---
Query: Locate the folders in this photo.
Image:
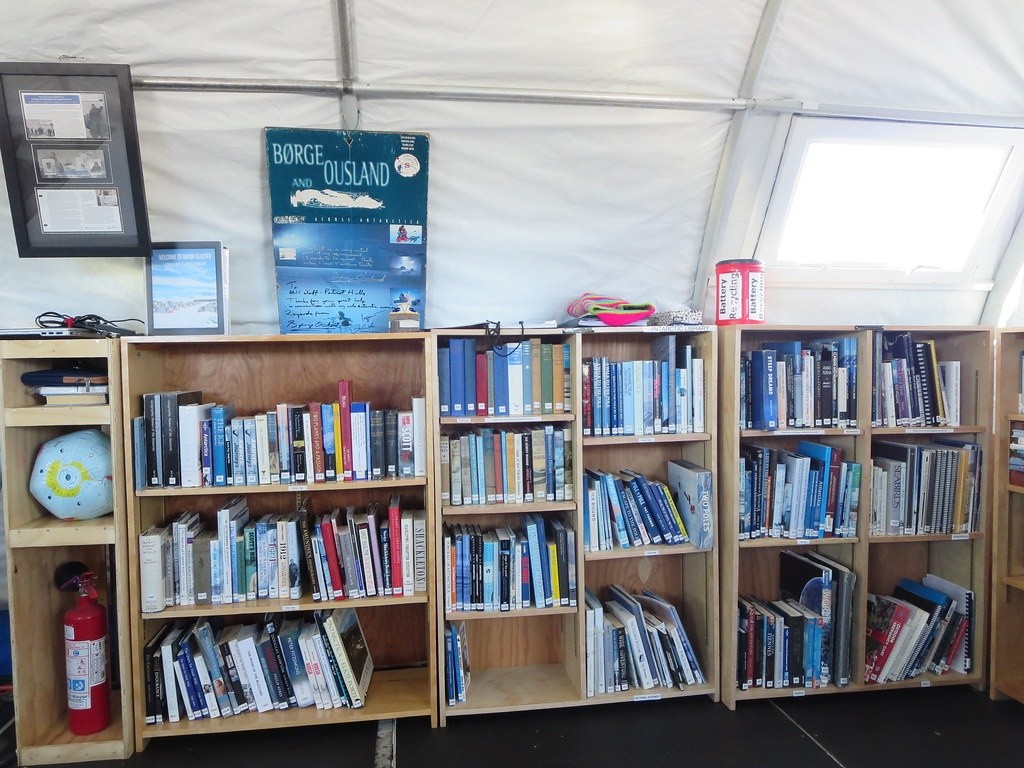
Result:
[438,338,476,416]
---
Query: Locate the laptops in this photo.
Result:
[0,328,136,339]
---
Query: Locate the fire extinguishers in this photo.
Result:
[61,572,112,735]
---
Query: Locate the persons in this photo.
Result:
[27,99,108,178]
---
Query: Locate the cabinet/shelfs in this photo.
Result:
[119,331,438,752]
[0,337,134,767]
[718,324,994,711]
[990,327,1024,704]
[429,324,730,728]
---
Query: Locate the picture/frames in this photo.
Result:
[145,241,224,336]
[0,62,153,258]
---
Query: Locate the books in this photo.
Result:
[868,438,984,538]
[439,334,572,417]
[442,512,578,615]
[865,571,974,685]
[1018,351,1024,413]
[144,607,376,727]
[737,549,854,692]
[583,457,716,553]
[1008,426,1024,488]
[137,491,428,613]
[739,438,862,545]
[439,422,574,504]
[582,334,710,438]
[38,385,109,407]
[133,374,427,488]
[737,333,859,431]
[584,583,705,698]
[420,318,558,331]
[445,620,471,708]
[872,328,964,429]
[556,312,653,329]
